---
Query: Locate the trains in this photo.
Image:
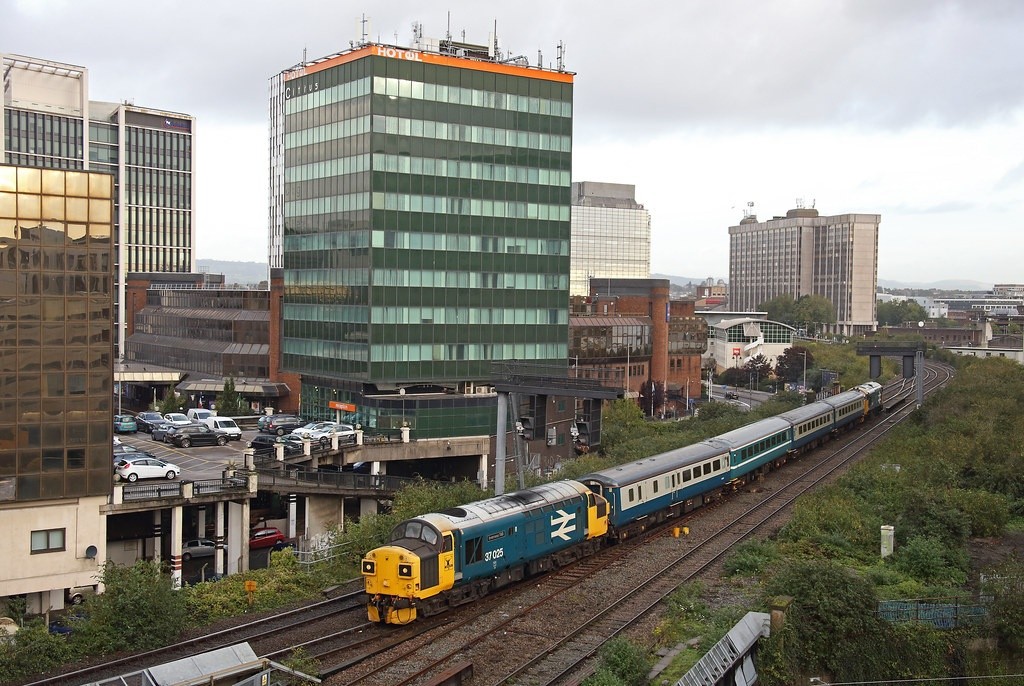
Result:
[359,381,883,626]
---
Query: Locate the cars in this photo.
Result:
[114,410,362,483]
[181,541,228,562]
[249,527,286,550]
